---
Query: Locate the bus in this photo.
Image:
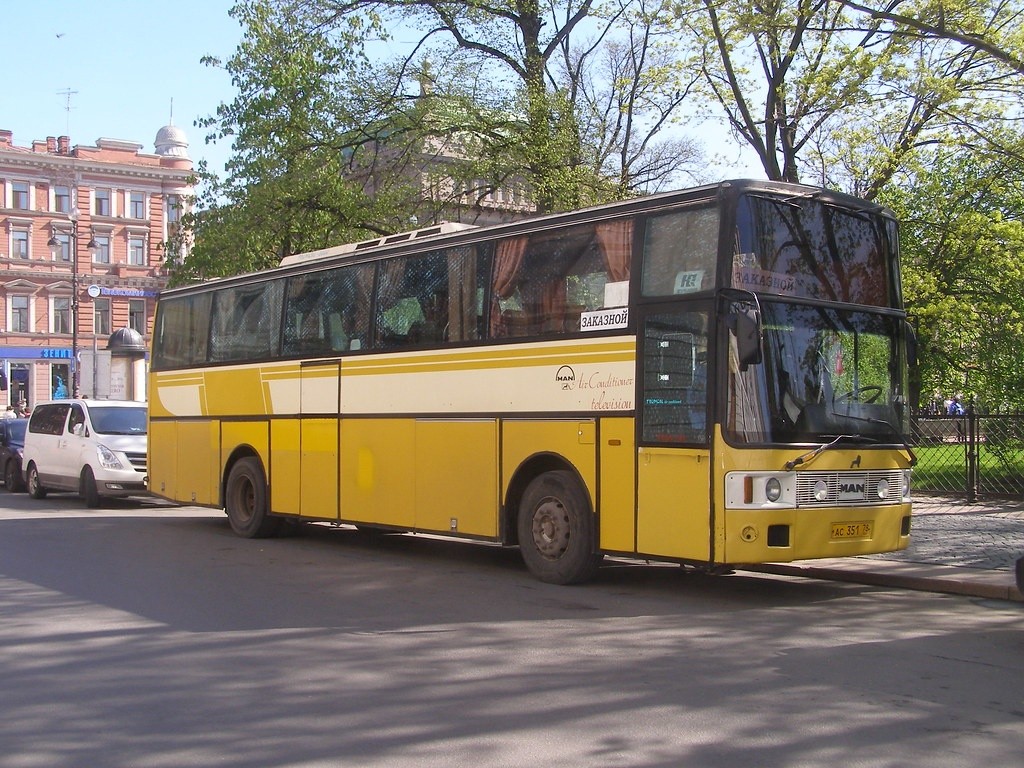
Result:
[144,179,921,586]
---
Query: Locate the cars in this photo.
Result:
[0,419,27,494]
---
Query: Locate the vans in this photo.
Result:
[22,400,148,507]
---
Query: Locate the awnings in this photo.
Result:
[107,327,149,352]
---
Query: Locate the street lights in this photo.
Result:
[46,206,102,400]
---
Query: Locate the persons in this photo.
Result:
[948,397,963,415]
[3,398,28,418]
